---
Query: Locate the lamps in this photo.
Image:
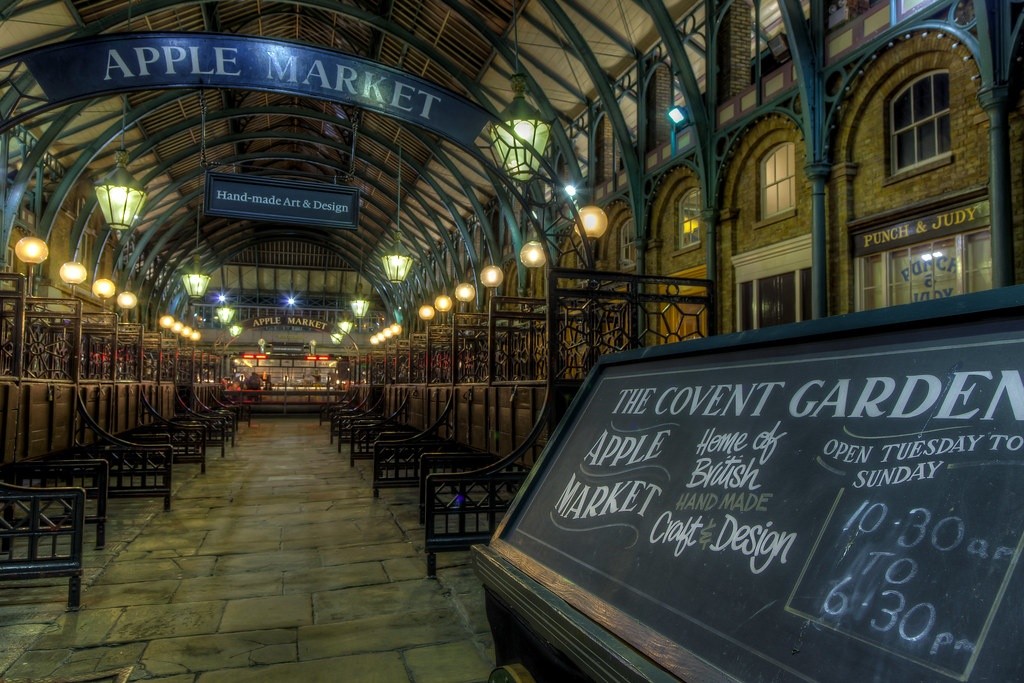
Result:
[419,304,435,332]
[349,229,371,319]
[383,327,394,343]
[337,277,355,335]
[390,323,402,339]
[455,282,476,313]
[189,331,201,347]
[171,321,183,340]
[572,205,607,267]
[480,265,504,297]
[490,1,556,181]
[92,93,150,230]
[668,105,688,126]
[182,126,213,298]
[160,315,174,338]
[228,322,243,337]
[330,333,343,345]
[92,279,116,313]
[521,240,547,297]
[180,325,192,346]
[381,135,414,283]
[60,261,87,299]
[216,261,236,323]
[14,237,49,297]
[434,294,453,322]
[117,290,137,323]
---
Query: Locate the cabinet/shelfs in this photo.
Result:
[322,268,718,581]
[0,272,252,611]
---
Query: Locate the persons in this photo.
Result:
[250,372,272,401]
